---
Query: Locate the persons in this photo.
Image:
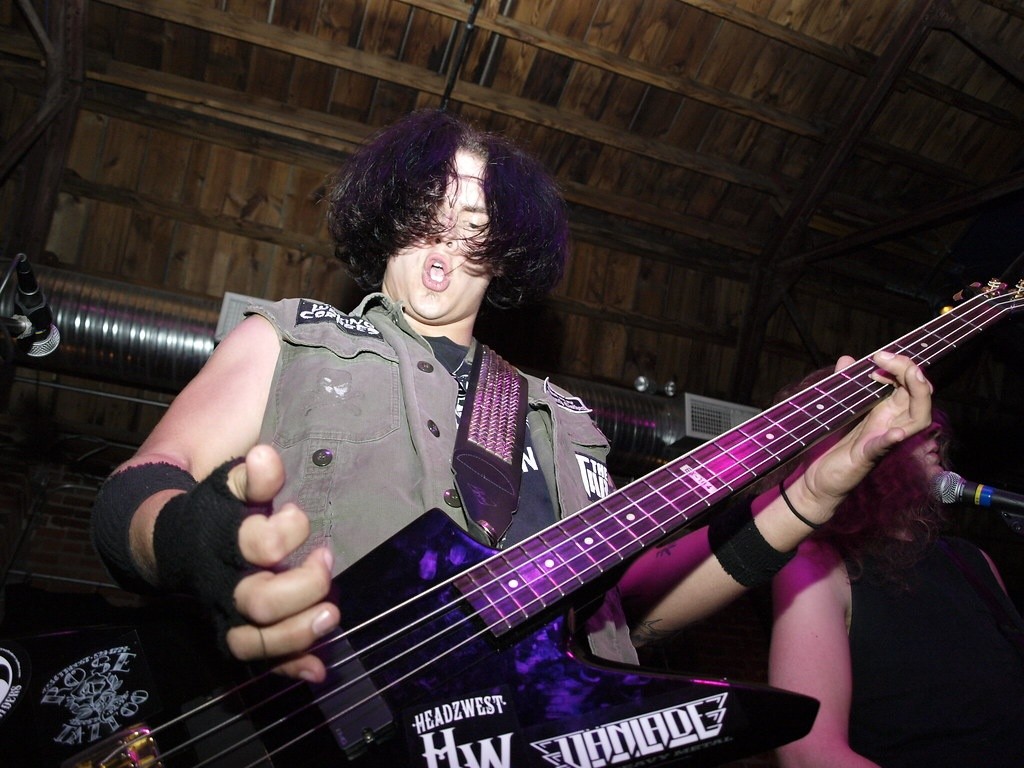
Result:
[769,406,1023,768]
[89,115,1024,768]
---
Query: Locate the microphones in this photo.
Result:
[930,471,1024,518]
[16,252,60,357]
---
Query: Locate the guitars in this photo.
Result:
[0,277,1024,768]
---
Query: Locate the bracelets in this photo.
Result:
[707,499,798,591]
[92,461,198,591]
[779,478,823,530]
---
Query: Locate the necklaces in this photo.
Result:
[451,357,468,392]
